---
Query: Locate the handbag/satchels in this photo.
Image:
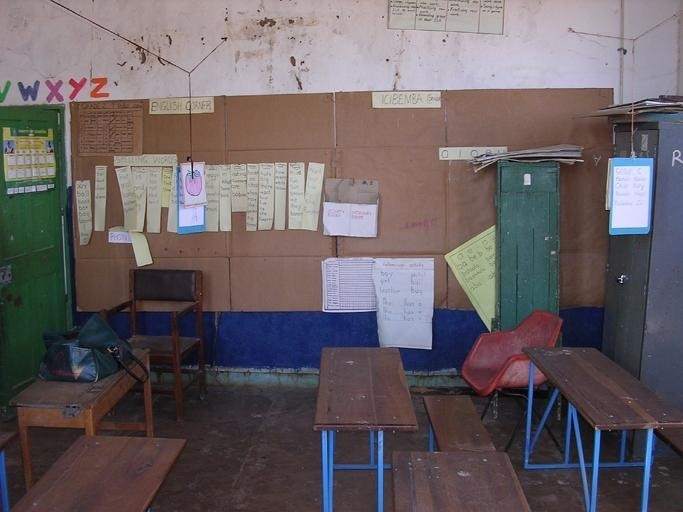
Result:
[35,312,153,385]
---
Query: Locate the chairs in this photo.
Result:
[97,268,210,423]
[459,310,567,456]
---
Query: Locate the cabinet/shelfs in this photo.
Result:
[602,110,681,405]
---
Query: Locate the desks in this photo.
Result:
[309,346,420,512]
[5,347,188,512]
[521,347,682,512]
[393,452,529,510]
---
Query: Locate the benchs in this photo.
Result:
[421,395,496,452]
